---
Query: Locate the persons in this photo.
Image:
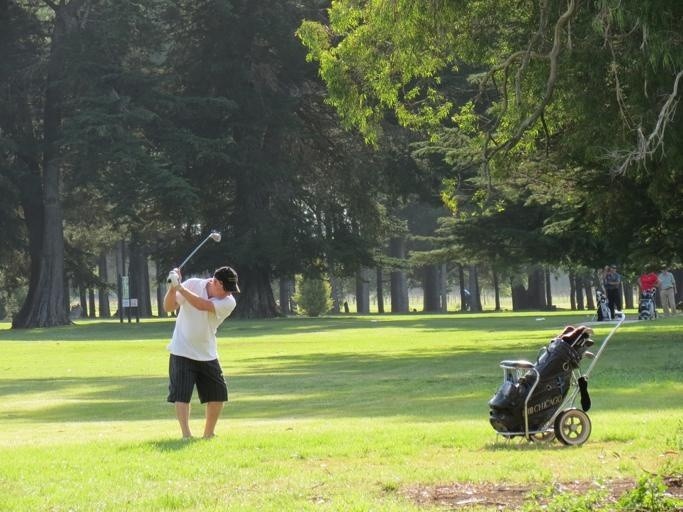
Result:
[637,265,662,292]
[603,264,624,321]
[163,266,240,440]
[658,266,678,318]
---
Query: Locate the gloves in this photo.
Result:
[169,268,182,286]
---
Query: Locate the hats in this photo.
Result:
[214,267,240,294]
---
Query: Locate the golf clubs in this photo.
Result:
[167,232,222,284]
[555,325,596,361]
